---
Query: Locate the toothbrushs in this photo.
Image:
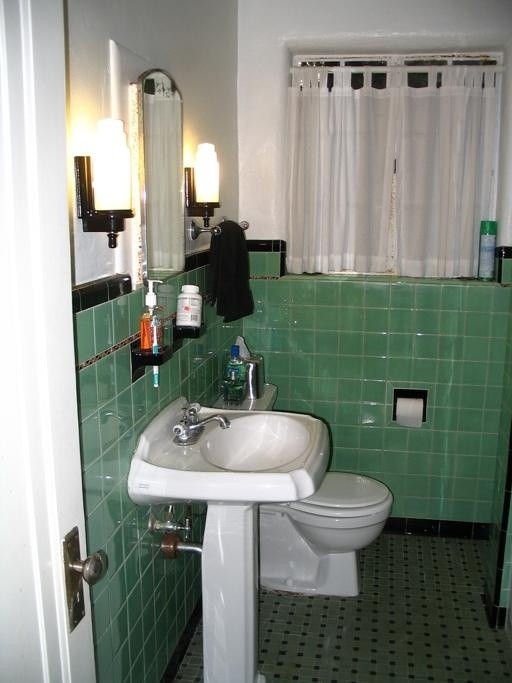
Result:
[151,316,159,387]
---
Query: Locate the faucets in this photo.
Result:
[189,414,230,430]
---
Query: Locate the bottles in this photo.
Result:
[176,284,203,328]
[224,345,247,401]
[157,284,176,327]
[218,368,246,406]
[477,220,497,283]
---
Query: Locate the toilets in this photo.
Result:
[213,382,393,597]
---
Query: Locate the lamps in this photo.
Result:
[182,142,222,229]
[74,119,135,249]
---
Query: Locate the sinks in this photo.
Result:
[127,395,330,504]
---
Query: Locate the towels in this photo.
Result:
[206,220,254,323]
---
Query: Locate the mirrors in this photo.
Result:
[129,68,187,286]
